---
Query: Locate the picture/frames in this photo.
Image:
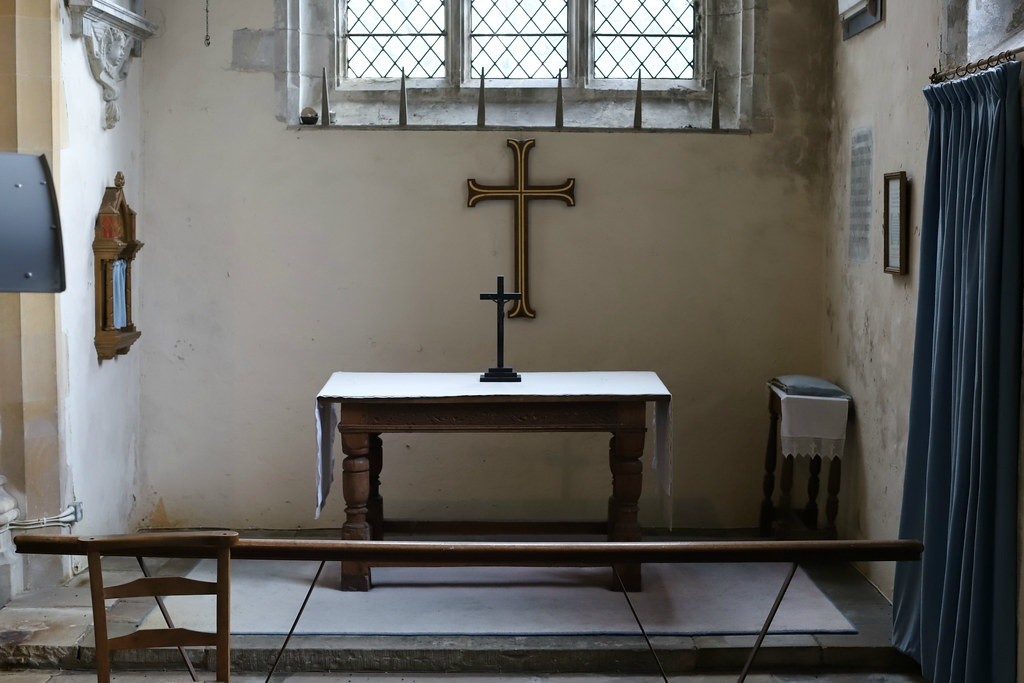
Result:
[883,171,909,276]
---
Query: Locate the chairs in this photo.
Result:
[78,531,241,683]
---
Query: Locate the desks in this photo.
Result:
[761,381,848,564]
[315,371,674,592]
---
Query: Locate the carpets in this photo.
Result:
[141,556,857,635]
